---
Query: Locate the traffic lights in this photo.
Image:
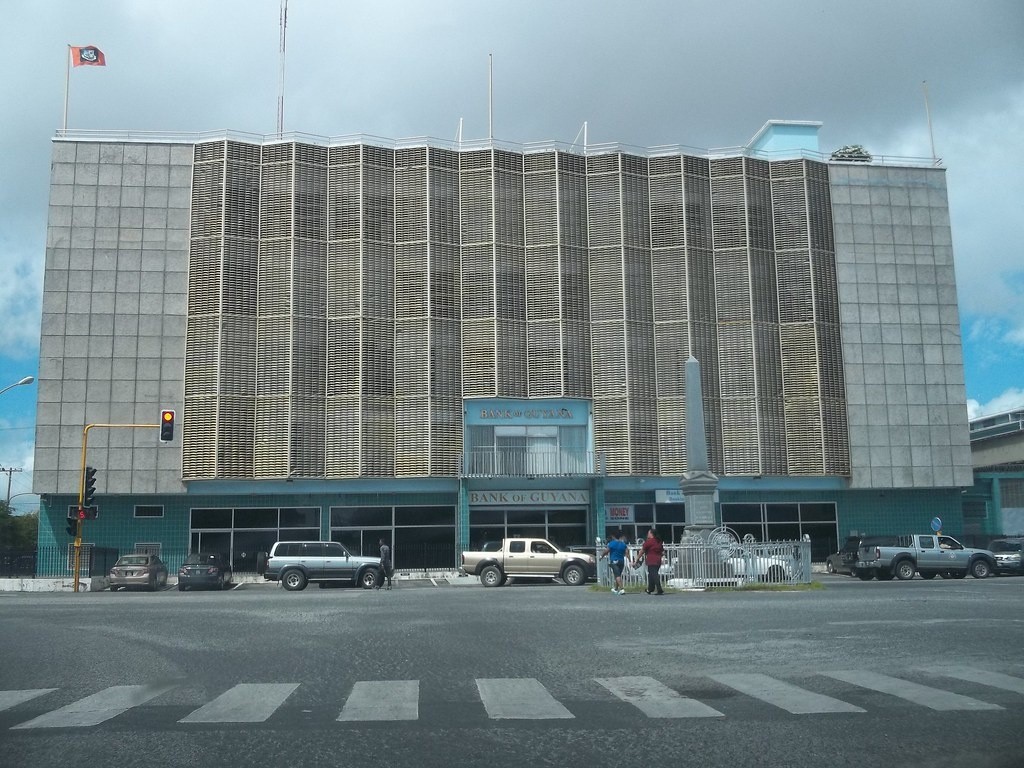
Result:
[83,467,96,508]
[160,410,173,441]
[66,516,78,535]
[80,508,98,519]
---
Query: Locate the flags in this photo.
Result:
[70,45,106,68]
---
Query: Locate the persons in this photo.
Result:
[373,539,392,590]
[633,529,666,595]
[598,530,632,595]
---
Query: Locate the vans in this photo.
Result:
[991,538,1024,575]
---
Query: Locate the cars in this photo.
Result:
[726,547,790,581]
[108,553,168,591]
[178,553,233,591]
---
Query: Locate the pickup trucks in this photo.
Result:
[462,538,597,587]
[874,535,996,580]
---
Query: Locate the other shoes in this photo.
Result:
[645,589,651,594]
[655,591,664,595]
[610,589,618,592]
[385,587,391,590]
[618,589,625,595]
[372,586,379,590]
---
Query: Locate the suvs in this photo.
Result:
[825,536,897,576]
[265,540,393,590]
